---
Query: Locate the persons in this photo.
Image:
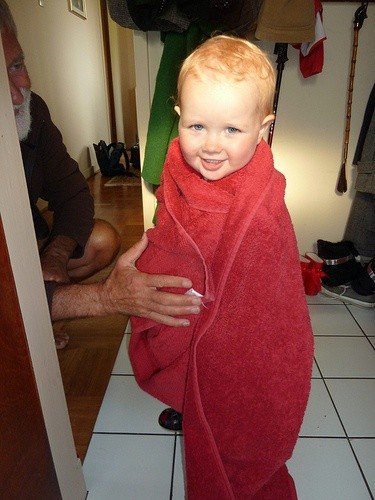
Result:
[136,35,277,427]
[0,0,202,327]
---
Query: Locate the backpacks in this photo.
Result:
[93,140,136,177]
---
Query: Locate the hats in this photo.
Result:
[255,0,316,43]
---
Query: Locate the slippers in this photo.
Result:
[159,408,183,431]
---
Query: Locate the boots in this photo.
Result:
[300,252,327,296]
